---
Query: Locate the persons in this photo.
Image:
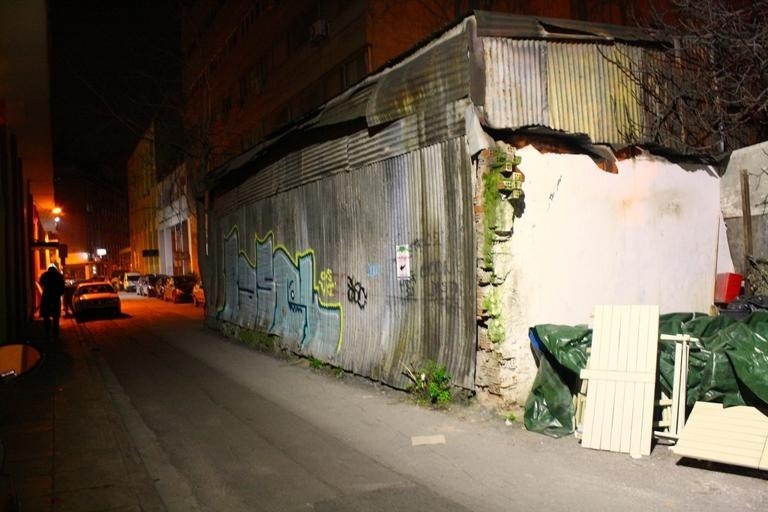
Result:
[39,267,66,338]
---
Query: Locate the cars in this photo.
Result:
[71,281,121,318]
[136,274,205,306]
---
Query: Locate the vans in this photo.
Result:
[123,273,140,292]
